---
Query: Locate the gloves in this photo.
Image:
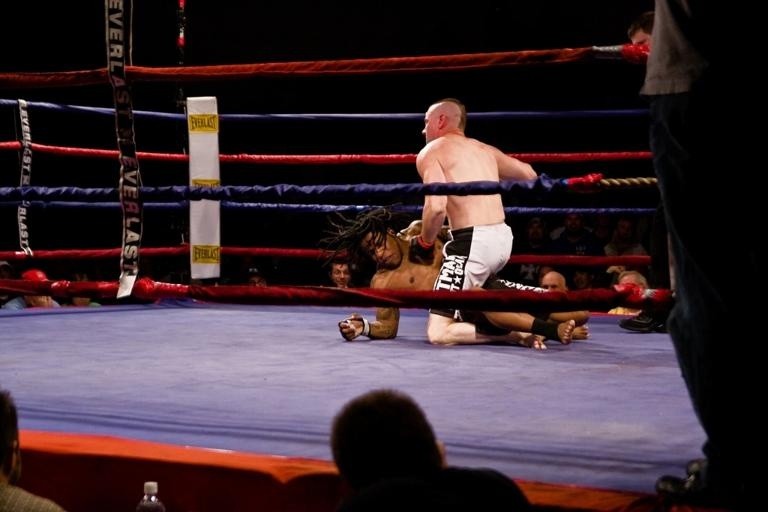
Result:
[409,235,435,266]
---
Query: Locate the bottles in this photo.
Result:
[136,479,165,512]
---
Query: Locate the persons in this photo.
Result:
[324,202,590,346]
[640,0,767,512]
[1,259,101,311]
[330,389,535,512]
[506,202,646,316]
[415,100,547,349]
[249,274,268,289]
[329,262,351,288]
[0,392,65,511]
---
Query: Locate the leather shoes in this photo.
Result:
[619,310,667,335]
[654,458,708,508]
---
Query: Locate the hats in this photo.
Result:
[21,269,49,282]
